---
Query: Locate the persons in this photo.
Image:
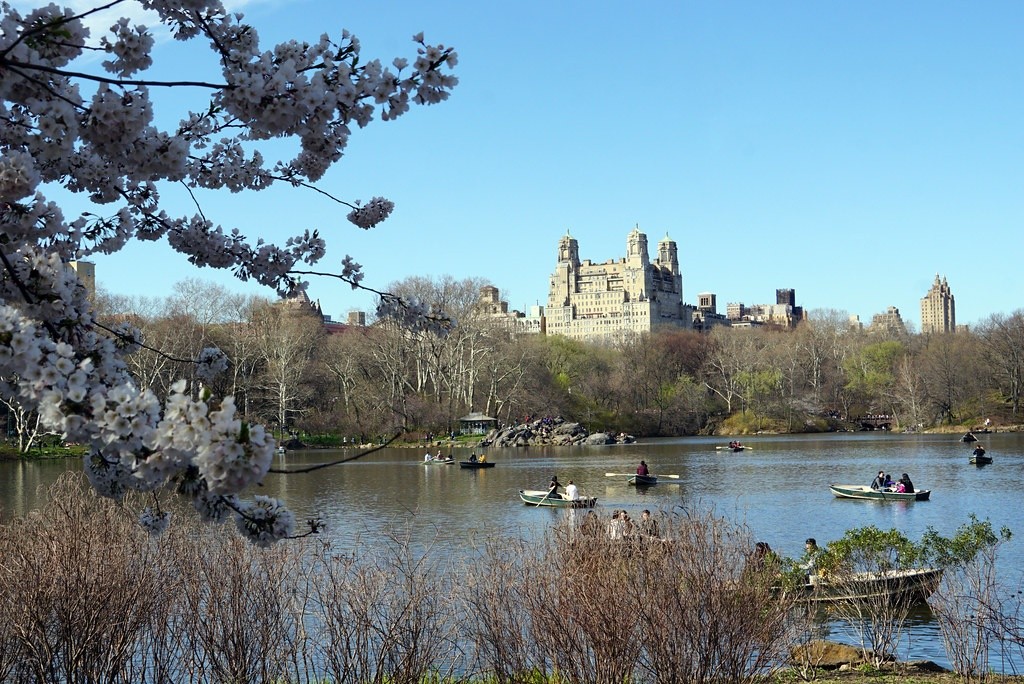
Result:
[289,429,299,439]
[450,430,455,440]
[984,418,990,427]
[359,434,363,445]
[342,436,347,446]
[872,471,915,493]
[582,510,659,540]
[965,432,972,437]
[729,440,741,448]
[435,450,443,459]
[424,452,432,462]
[279,445,284,450]
[515,417,519,424]
[973,445,985,457]
[636,461,649,475]
[564,480,579,500]
[571,426,586,437]
[469,452,477,462]
[542,414,554,435]
[478,454,487,463]
[790,538,824,583]
[751,542,782,586]
[351,437,356,445]
[425,431,434,443]
[970,427,987,432]
[549,476,563,499]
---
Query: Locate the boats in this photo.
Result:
[969,455,993,464]
[828,484,931,501]
[519,489,598,508]
[626,474,657,486]
[459,461,496,468]
[723,446,745,452]
[722,566,946,610]
[419,459,455,465]
[278,448,287,453]
[960,431,979,443]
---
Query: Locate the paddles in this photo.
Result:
[605,472,680,479]
[884,487,898,492]
[959,433,967,441]
[716,446,753,450]
[536,486,555,507]
[969,433,979,441]
[421,455,436,465]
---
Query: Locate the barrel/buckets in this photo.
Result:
[809,575,820,583]
